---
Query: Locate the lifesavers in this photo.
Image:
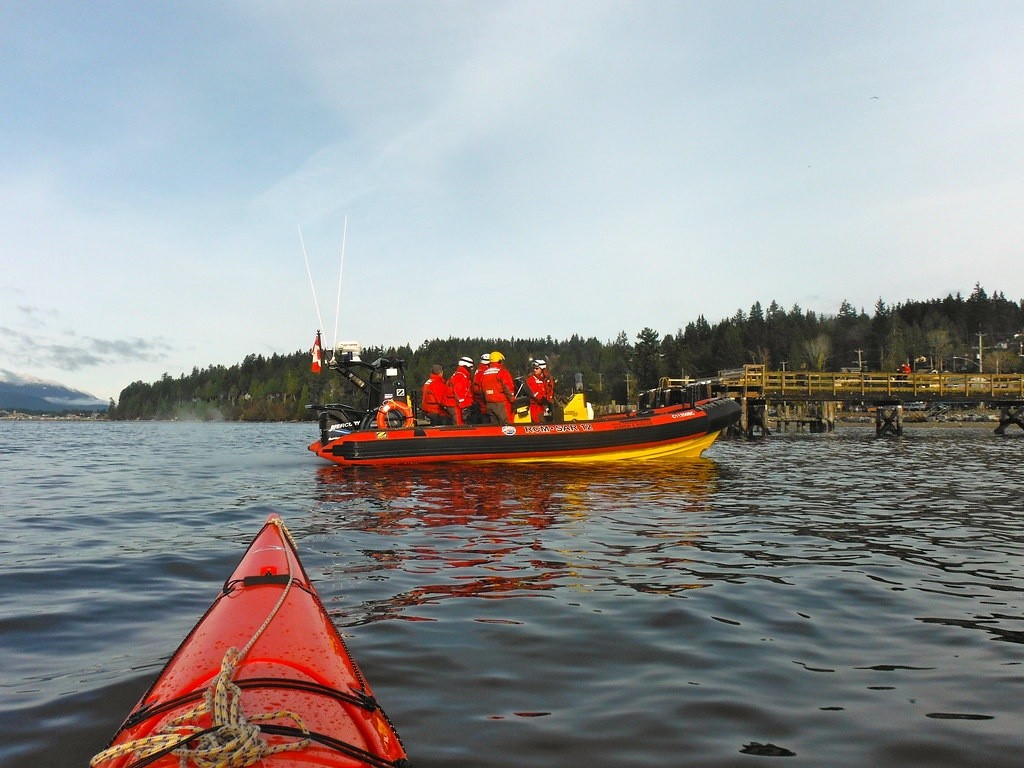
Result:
[376,400,414,429]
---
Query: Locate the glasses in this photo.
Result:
[533,367,540,370]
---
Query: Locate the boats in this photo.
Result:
[296,214,743,471]
[86,511,413,768]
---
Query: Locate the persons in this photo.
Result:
[477,351,515,424]
[524,360,554,424]
[442,357,476,425]
[901,362,911,387]
[473,353,490,424]
[421,365,452,426]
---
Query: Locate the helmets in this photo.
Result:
[479,353,490,364]
[531,359,547,369]
[489,351,505,362]
[458,356,473,367]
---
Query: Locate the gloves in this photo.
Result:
[541,397,548,406]
[547,403,552,414]
[510,402,517,414]
